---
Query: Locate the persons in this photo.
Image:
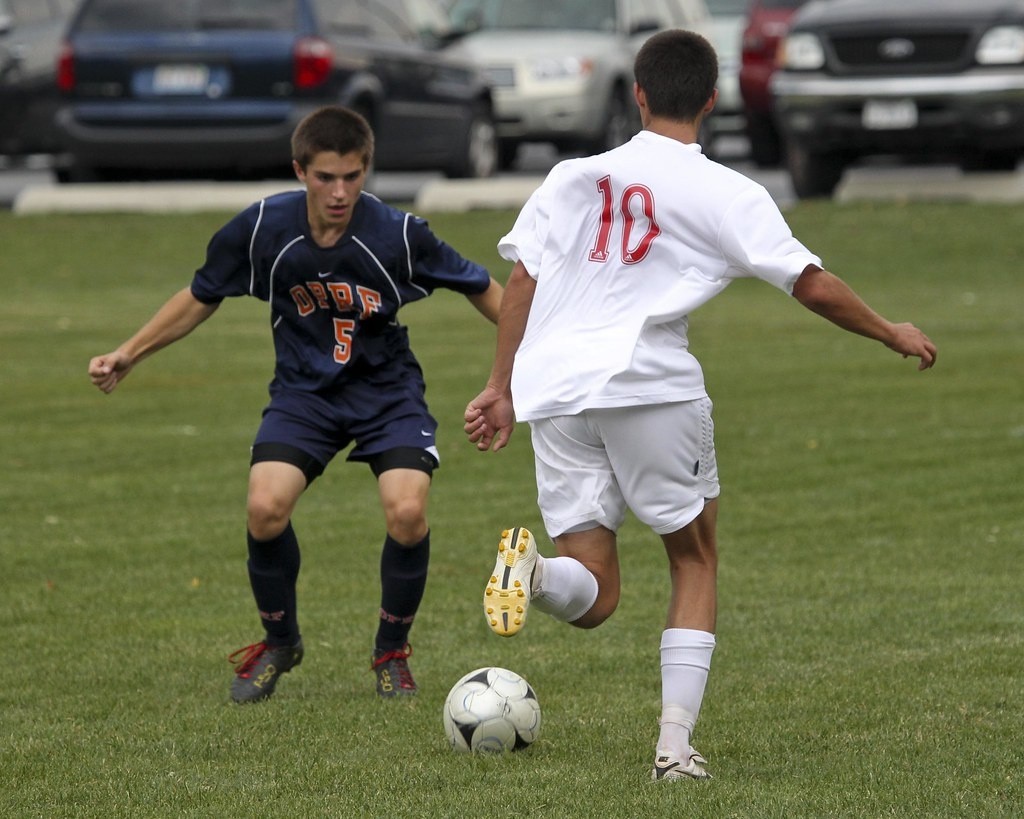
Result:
[462,31,940,781]
[86,105,506,706]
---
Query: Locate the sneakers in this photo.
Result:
[231,637,304,704]
[651,746,714,782]
[371,647,419,697]
[483,527,536,637]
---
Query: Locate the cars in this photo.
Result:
[49,1,500,179]
[742,1,802,158]
[775,0,1024,201]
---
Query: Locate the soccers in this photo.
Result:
[443,667,541,753]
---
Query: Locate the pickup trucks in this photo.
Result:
[450,1,749,172]
